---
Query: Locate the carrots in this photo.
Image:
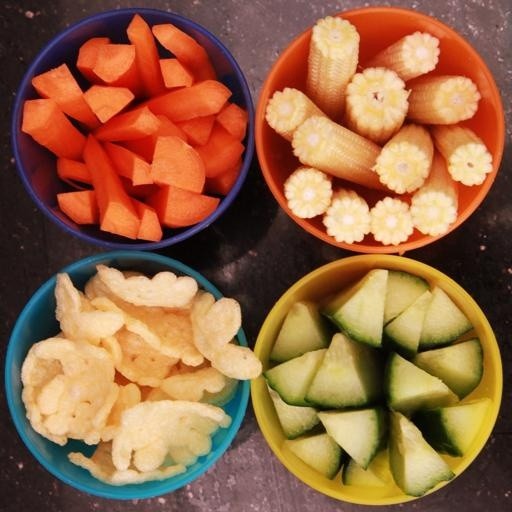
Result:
[21,13,249,244]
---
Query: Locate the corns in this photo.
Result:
[266,15,493,247]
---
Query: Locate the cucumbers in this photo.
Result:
[261,268,491,497]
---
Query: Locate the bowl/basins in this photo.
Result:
[253,5,507,254]
[10,5,256,252]
[4,250,254,504]
[250,254,505,507]
